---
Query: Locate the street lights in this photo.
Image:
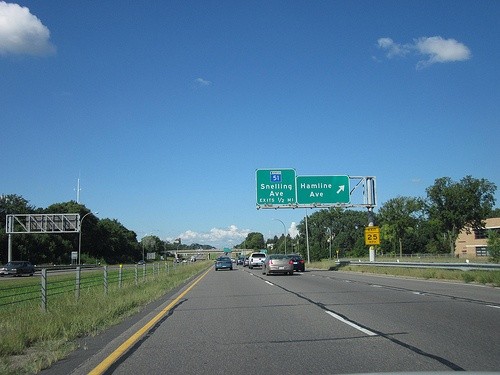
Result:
[272,218,287,255]
[78,211,99,269]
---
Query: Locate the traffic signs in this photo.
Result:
[296,176,350,204]
[255,168,297,206]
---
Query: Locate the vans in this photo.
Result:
[248,252,266,271]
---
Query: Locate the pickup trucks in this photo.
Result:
[214,256,233,271]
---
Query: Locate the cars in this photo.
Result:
[261,255,295,276]
[137,256,202,266]
[286,254,305,272]
[231,256,249,268]
[0,260,35,278]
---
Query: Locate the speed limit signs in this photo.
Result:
[365,226,380,245]
